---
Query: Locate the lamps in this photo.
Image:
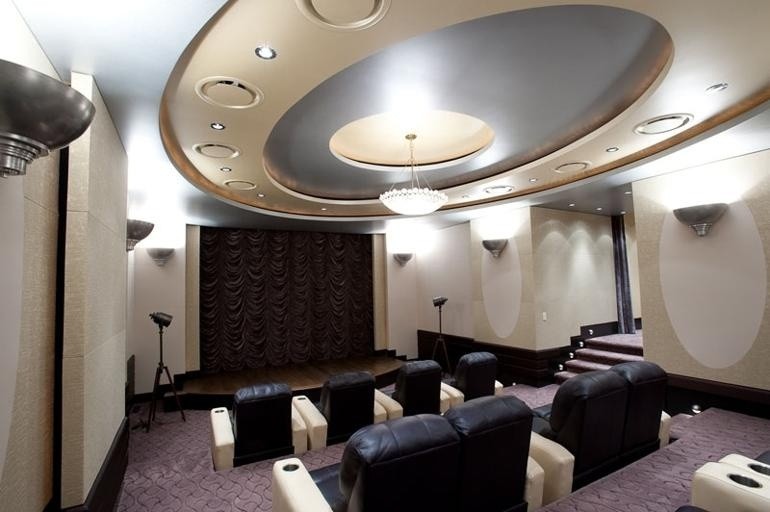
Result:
[431,295,448,308]
[147,311,175,328]
[0,58,97,179]
[394,254,412,267]
[378,132,449,216]
[482,240,509,258]
[127,219,155,250]
[673,203,731,235]
[147,248,174,266]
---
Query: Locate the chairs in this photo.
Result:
[440,352,504,410]
[208,381,308,473]
[292,370,389,450]
[374,359,452,422]
[528,351,673,507]
[272,391,546,510]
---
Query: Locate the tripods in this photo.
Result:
[429,306,451,378]
[146,327,186,433]
